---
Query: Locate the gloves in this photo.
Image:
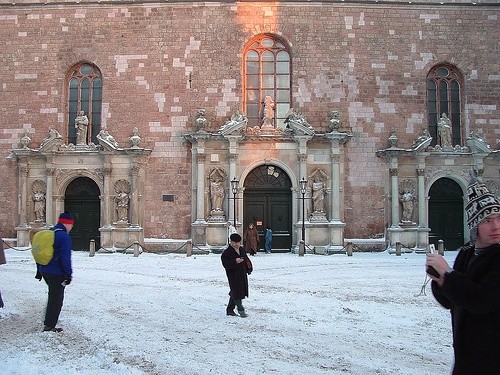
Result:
[64,275,72,285]
[35,270,42,282]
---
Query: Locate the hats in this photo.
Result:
[58,212,75,225]
[465,175,500,247]
[230,233,241,242]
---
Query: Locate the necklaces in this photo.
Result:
[425,178,500,375]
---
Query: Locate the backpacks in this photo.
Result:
[31,228,63,266]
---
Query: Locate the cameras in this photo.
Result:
[426,265,440,278]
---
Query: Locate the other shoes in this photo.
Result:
[227,312,238,316]
[239,314,248,317]
[44,328,63,332]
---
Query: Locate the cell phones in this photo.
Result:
[240,257,244,259]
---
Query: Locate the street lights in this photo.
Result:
[298,176,308,254]
[231,177,239,233]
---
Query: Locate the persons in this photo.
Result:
[32,189,45,220]
[220,233,253,318]
[115,189,128,220]
[74,110,89,144]
[288,107,313,129]
[413,126,432,148]
[0,235,6,307]
[219,110,243,131]
[438,113,452,146]
[226,222,272,255]
[312,176,325,212]
[40,126,63,148]
[210,177,225,208]
[98,126,118,147]
[468,128,491,149]
[264,96,274,126]
[399,188,414,221]
[29,212,74,333]
